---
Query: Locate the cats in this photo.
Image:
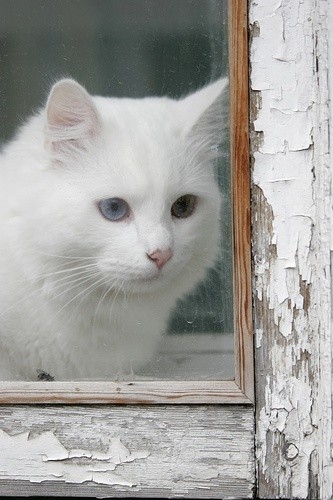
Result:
[0,76,231,380]
